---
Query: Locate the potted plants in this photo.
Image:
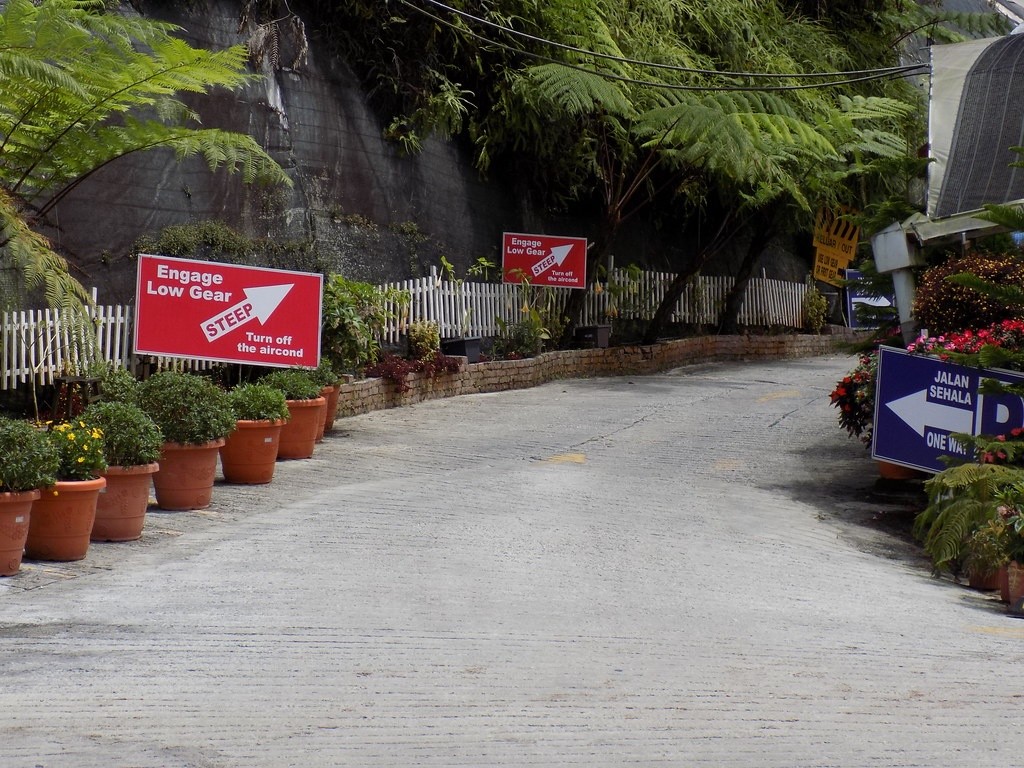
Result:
[435,254,496,364]
[73,402,161,545]
[126,368,237,514]
[217,380,292,487]
[912,432,1024,616]
[259,356,346,461]
[1,416,60,578]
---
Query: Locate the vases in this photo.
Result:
[875,457,916,481]
[574,324,611,350]
[24,477,108,562]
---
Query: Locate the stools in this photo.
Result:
[50,372,104,422]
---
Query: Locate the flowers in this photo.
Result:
[29,418,109,497]
[825,349,877,447]
